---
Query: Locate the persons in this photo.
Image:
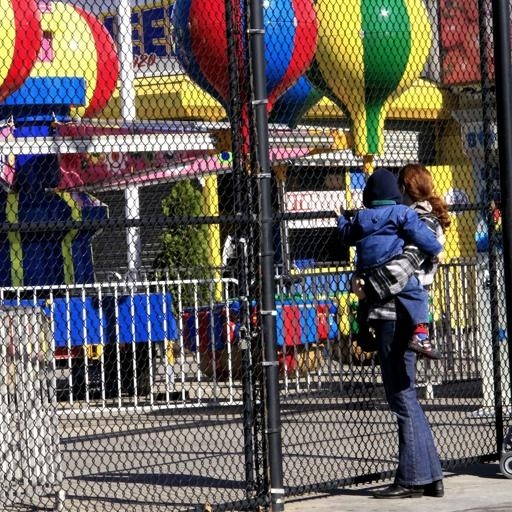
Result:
[333,169,447,361]
[349,163,452,500]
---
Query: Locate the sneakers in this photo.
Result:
[408,338,442,359]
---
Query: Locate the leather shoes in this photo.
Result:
[424,477,444,497]
[373,481,424,499]
